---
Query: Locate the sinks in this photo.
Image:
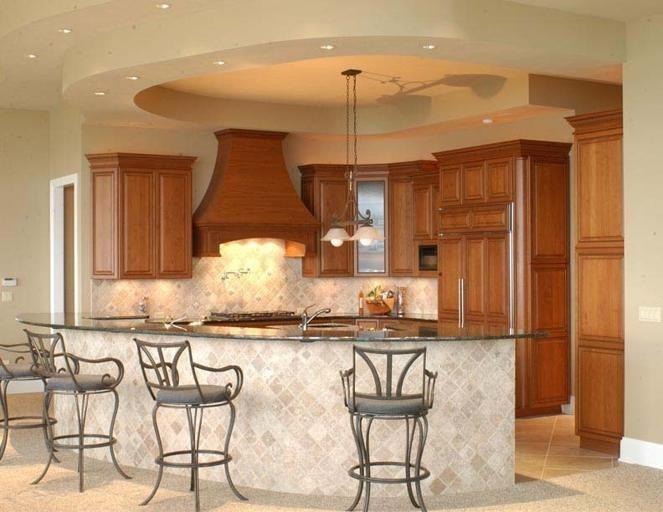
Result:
[306,324,349,328]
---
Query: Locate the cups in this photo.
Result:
[192,312,201,328]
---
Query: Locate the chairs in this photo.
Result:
[339,345,436,511]
[24,329,133,494]
[132,337,249,511]
[1,343,62,463]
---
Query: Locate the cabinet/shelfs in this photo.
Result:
[562,105,625,460]
[413,161,438,278]
[296,161,415,278]
[431,139,570,418]
[84,152,198,280]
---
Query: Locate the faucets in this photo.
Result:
[300,303,330,329]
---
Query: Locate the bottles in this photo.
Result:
[139,296,147,313]
[358,286,364,317]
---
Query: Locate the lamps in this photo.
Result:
[317,69,387,247]
